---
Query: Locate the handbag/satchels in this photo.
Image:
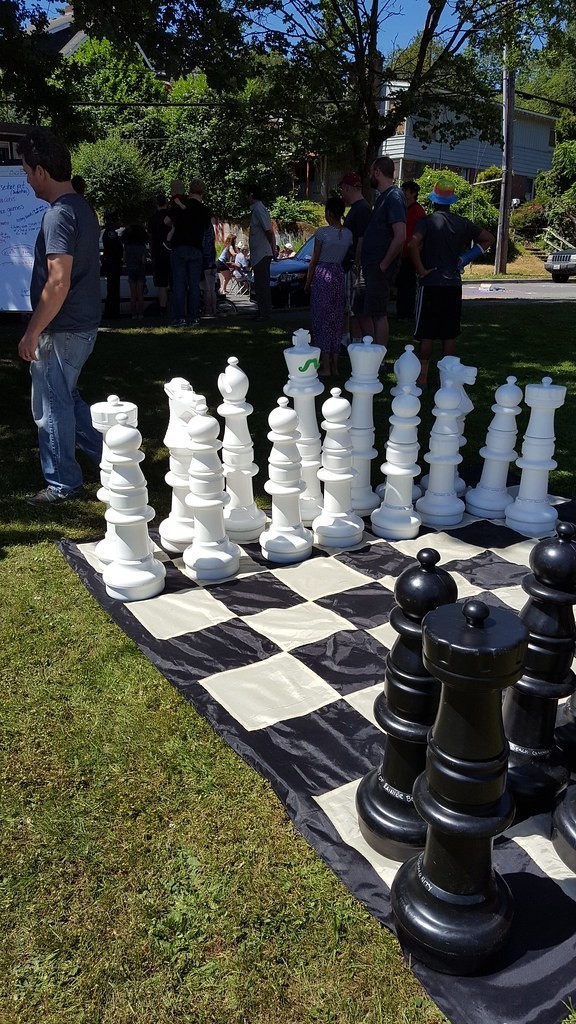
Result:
[102,248,119,273]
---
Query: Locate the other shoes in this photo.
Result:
[131,314,138,319]
[163,241,173,250]
[83,470,100,482]
[137,313,144,319]
[201,315,217,318]
[381,362,388,369]
[26,485,85,506]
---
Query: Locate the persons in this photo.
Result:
[232,246,250,295]
[163,181,187,251]
[284,246,296,258]
[352,157,406,366]
[217,235,247,297]
[396,181,426,322]
[201,261,216,320]
[275,245,284,259]
[147,195,172,319]
[18,127,103,506]
[102,211,124,320]
[337,172,371,354]
[246,185,279,322]
[407,178,495,391]
[304,197,353,376]
[71,175,99,226]
[164,180,211,327]
[120,224,150,319]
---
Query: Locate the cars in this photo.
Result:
[248,233,317,310]
[544,247,576,283]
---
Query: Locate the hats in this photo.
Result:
[235,241,244,249]
[400,180,420,191]
[337,171,362,186]
[285,243,293,249]
[172,182,185,193]
[427,180,458,205]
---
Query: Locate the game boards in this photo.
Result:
[54,448,576,1024]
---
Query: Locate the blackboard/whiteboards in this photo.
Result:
[0,159,51,313]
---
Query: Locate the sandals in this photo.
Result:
[256,315,270,321]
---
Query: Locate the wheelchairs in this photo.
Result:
[229,266,253,297]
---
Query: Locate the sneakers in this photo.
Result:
[168,318,186,327]
[184,318,201,325]
[416,379,428,390]
